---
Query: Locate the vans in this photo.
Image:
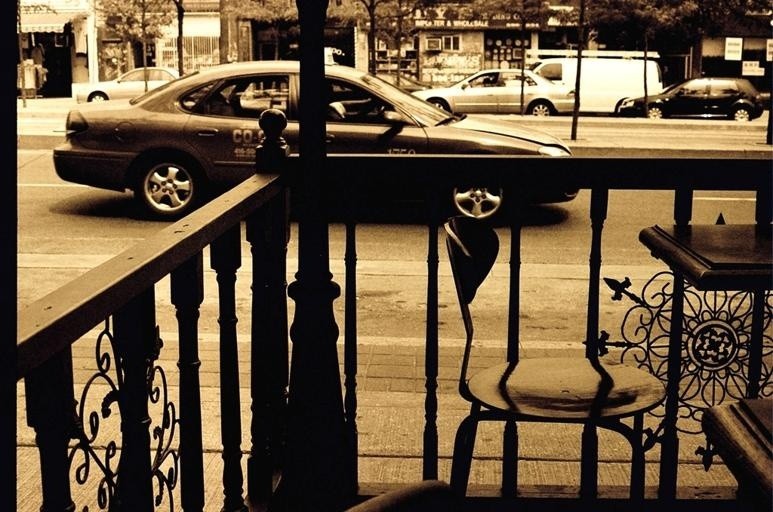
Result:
[532,57,663,113]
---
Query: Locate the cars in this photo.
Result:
[412,68,574,116]
[53,61,579,221]
[70,67,179,103]
[614,76,764,122]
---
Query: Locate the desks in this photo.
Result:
[639,221,773,399]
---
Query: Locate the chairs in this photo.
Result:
[443,213,666,512]
